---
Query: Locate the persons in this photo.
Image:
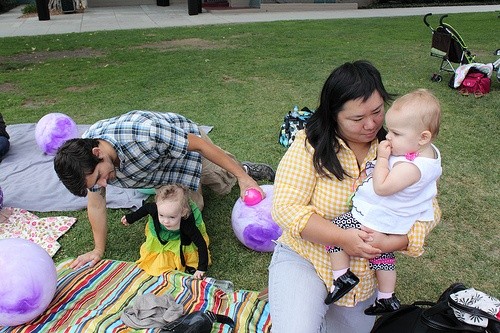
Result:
[0,111,11,162]
[325,87,443,315]
[53,109,264,270]
[119,183,211,283]
[268,58,440,333]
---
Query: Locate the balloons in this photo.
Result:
[34,112,79,156]
[231,183,287,253]
[0,236,58,326]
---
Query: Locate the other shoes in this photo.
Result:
[324,267,361,305]
[364,293,402,315]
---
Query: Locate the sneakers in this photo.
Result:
[242,161,275,182]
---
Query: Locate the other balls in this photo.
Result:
[35,113,78,156]
[244,190,262,206]
[0,238,57,327]
[231,185,283,251]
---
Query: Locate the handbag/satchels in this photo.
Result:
[458,72,491,99]
[413,282,500,333]
[278,104,315,147]
[158,310,235,333]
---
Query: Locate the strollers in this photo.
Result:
[423,12,477,83]
